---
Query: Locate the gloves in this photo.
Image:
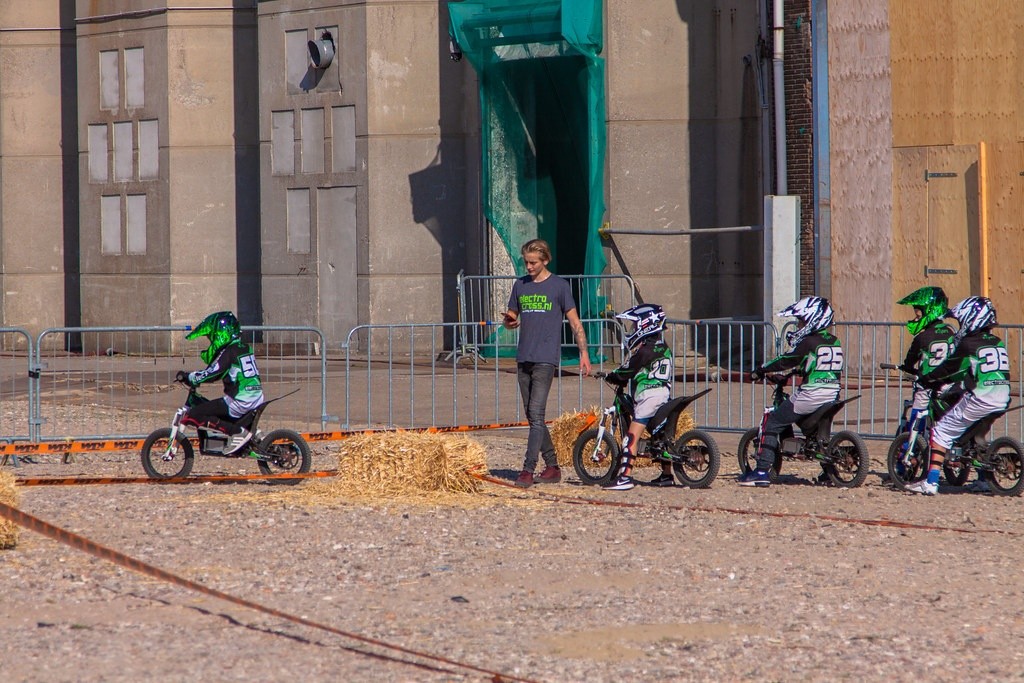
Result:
[176,370,192,387]
[749,365,765,381]
[915,375,928,392]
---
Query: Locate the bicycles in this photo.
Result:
[573,372,721,491]
[737,367,870,488]
[876,362,1024,497]
[140,368,312,484]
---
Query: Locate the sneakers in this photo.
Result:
[223,426,252,455]
[533,467,561,483]
[602,475,634,489]
[904,478,939,495]
[737,469,771,486]
[818,473,836,487]
[651,472,675,486]
[515,470,533,488]
[966,480,991,492]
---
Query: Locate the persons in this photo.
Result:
[894,288,1011,496]
[500,239,592,489]
[601,302,675,490]
[173,310,264,455]
[734,296,843,486]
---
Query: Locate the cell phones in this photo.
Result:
[501,312,517,322]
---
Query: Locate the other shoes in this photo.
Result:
[882,474,904,487]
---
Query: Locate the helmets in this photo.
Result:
[776,295,834,348]
[896,286,948,336]
[185,311,243,365]
[944,296,998,347]
[615,304,668,350]
